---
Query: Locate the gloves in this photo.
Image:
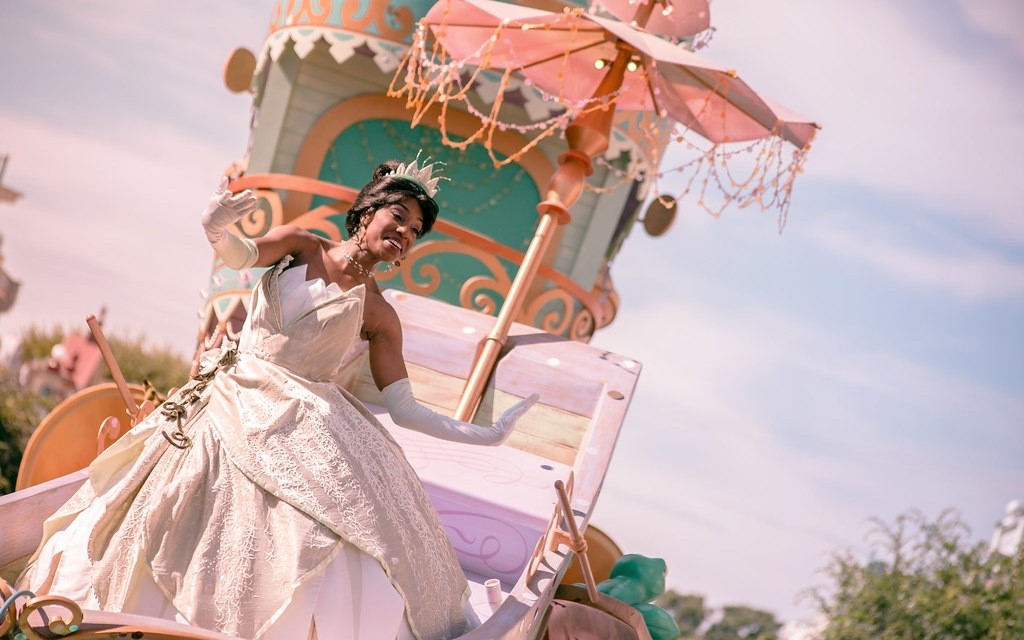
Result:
[202,176,260,270]
[379,376,539,446]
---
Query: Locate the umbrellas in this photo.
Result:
[384,0,821,418]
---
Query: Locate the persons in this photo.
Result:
[141,159,543,640]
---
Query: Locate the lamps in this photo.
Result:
[626,58,644,72]
[594,58,614,70]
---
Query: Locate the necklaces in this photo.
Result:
[340,239,376,278]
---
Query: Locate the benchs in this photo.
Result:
[363,400,573,588]
